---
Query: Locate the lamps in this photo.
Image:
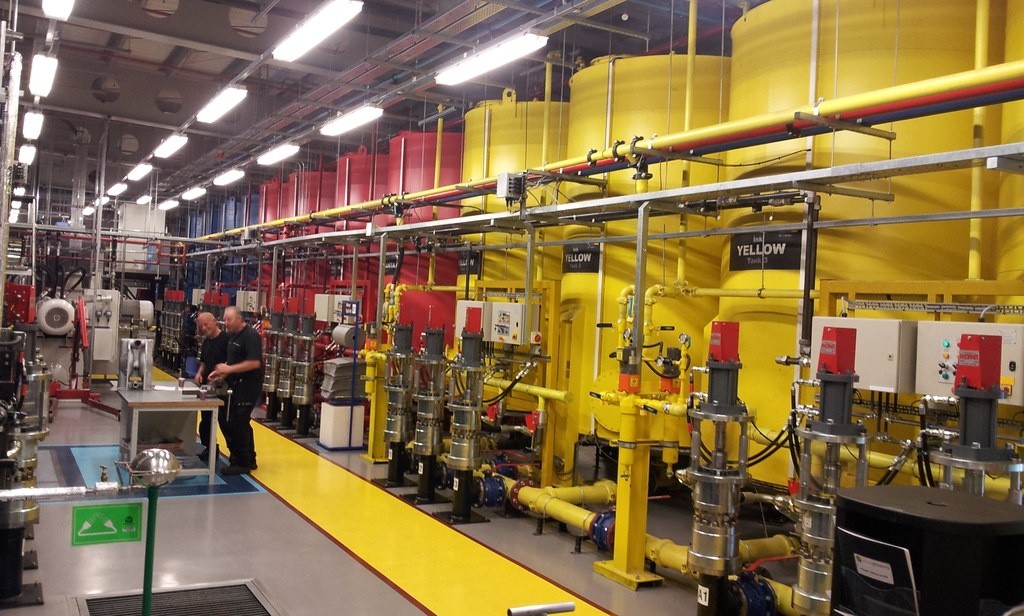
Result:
[7,0,552,225]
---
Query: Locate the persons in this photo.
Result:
[193,311,233,461]
[206,305,263,476]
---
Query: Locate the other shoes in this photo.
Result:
[247,462,257,470]
[220,463,250,476]
[198,444,219,461]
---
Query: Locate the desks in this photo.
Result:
[108,378,225,487]
